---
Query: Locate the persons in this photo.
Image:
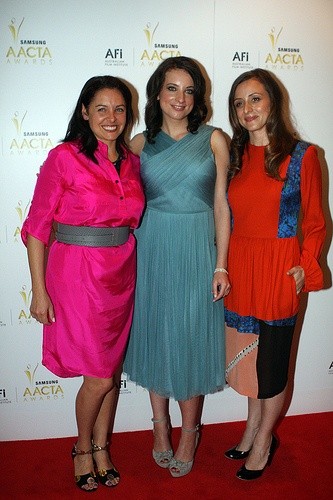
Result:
[129,55,232,478]
[226,68,326,481]
[21,76,144,490]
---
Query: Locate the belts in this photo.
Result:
[55,224,130,247]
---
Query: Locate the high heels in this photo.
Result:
[71,440,98,492]
[224,443,251,459]
[236,434,276,481]
[91,437,120,488]
[169,424,200,478]
[151,417,173,468]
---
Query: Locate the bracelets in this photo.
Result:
[214,268,228,274]
